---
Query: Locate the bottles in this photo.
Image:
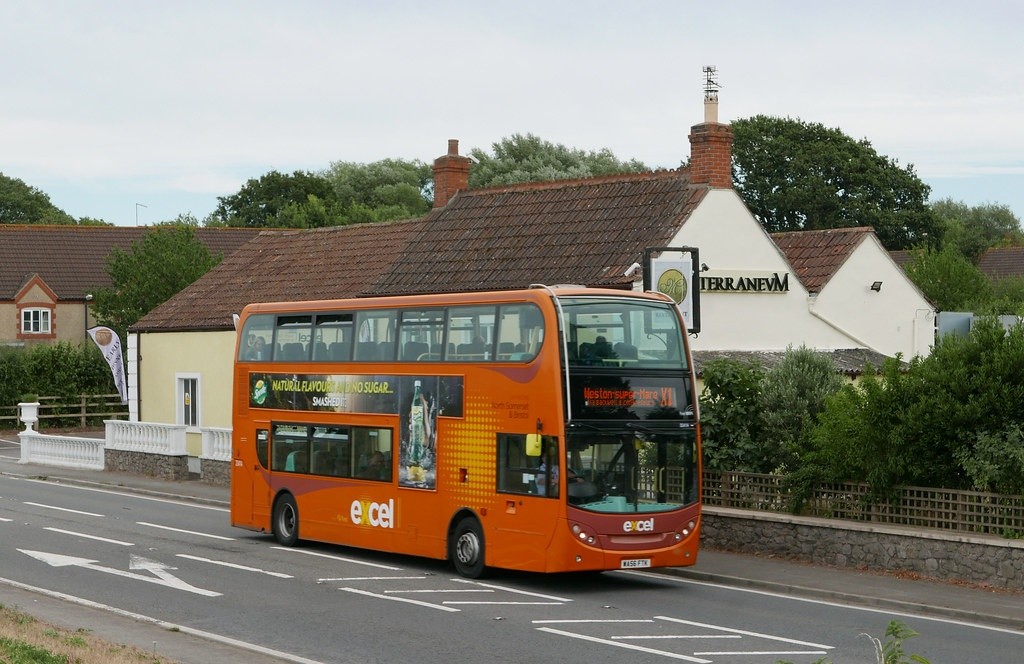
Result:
[408,380,424,460]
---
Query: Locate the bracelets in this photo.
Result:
[426,432,434,452]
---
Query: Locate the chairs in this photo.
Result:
[284,449,391,480]
[263,341,639,364]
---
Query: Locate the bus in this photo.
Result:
[231,284,703,579]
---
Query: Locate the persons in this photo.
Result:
[534,448,584,496]
[472,335,486,350]
[365,451,389,481]
[408,394,435,454]
[244,336,266,361]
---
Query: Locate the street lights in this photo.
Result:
[84,294,93,349]
